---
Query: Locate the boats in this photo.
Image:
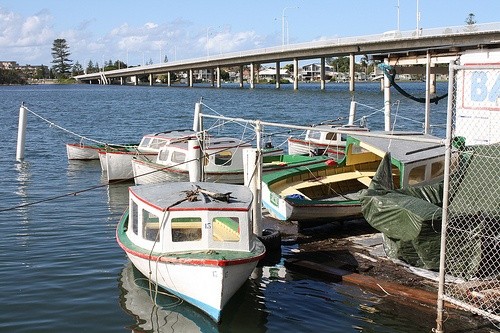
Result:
[259,130,461,221]
[66,122,372,186]
[114,180,268,324]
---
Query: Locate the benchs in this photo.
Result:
[272,168,378,200]
